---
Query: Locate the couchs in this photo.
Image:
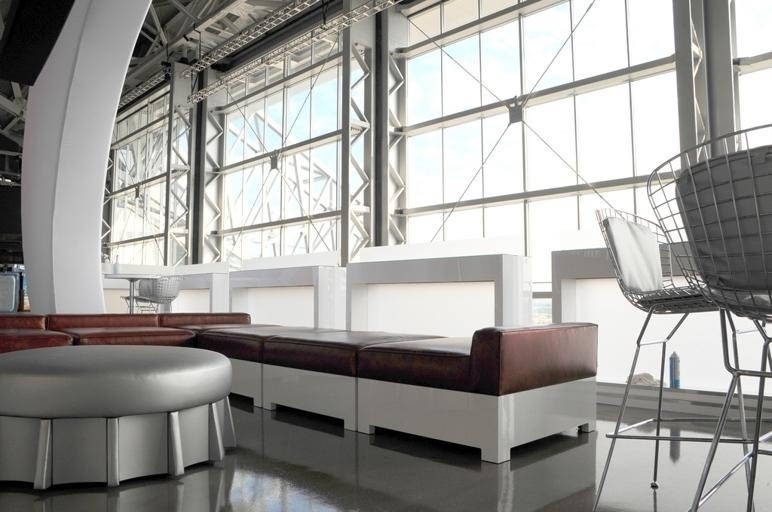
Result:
[2,312,251,349]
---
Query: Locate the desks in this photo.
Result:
[105,271,159,314]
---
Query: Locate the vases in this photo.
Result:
[114,255,120,273]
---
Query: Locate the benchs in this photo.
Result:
[170,322,599,464]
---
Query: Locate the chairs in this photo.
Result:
[594,204,772,512]
[136,275,181,315]
[645,125,772,510]
[121,278,160,312]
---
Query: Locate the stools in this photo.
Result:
[2,346,241,492]
